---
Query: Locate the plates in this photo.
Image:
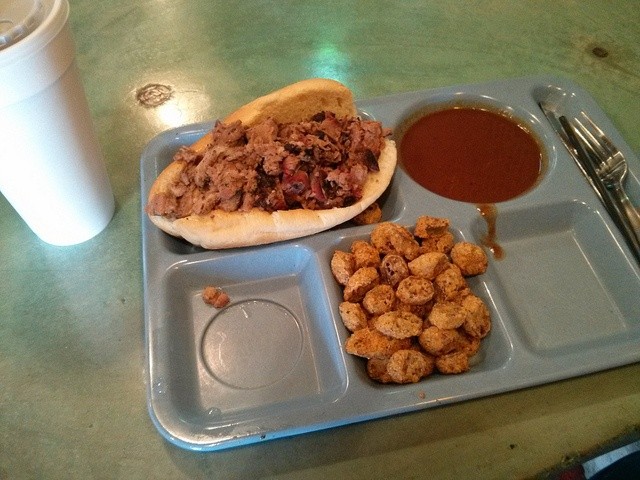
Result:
[139,75,640,453]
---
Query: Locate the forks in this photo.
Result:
[560,110,639,246]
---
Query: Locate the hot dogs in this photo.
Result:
[141,76,398,250]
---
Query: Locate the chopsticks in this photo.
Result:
[558,114,640,260]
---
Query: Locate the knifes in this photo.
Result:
[538,99,640,257]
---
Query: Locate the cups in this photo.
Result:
[0,0,116,247]
